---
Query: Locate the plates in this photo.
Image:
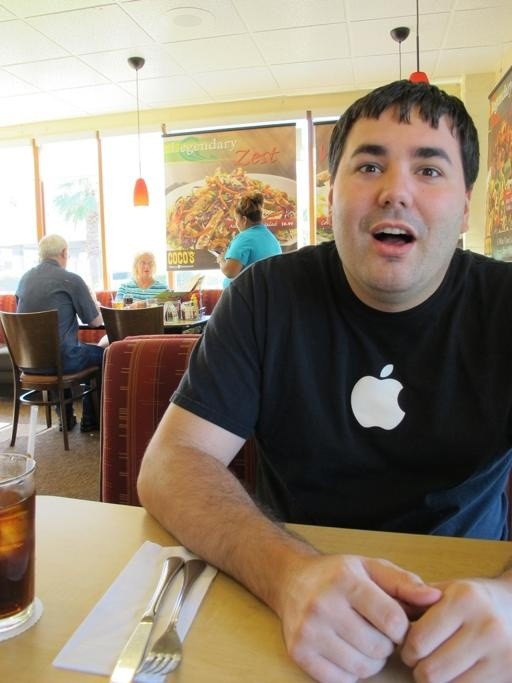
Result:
[165,172,300,249]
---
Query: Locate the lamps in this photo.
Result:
[410,3,428,86]
[127,56,152,208]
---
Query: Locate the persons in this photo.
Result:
[216,193,282,288]
[15,233,105,432]
[136,80,512,683]
[116,251,171,303]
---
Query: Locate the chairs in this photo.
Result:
[99,307,165,345]
[1,307,102,451]
[99,333,204,505]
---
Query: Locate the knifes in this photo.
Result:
[107,554,183,683]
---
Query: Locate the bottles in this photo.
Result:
[182,285,202,321]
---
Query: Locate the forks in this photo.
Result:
[132,559,208,683]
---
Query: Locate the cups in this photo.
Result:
[110,291,133,310]
[0,452,43,629]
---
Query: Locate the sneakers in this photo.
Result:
[58,413,95,432]
